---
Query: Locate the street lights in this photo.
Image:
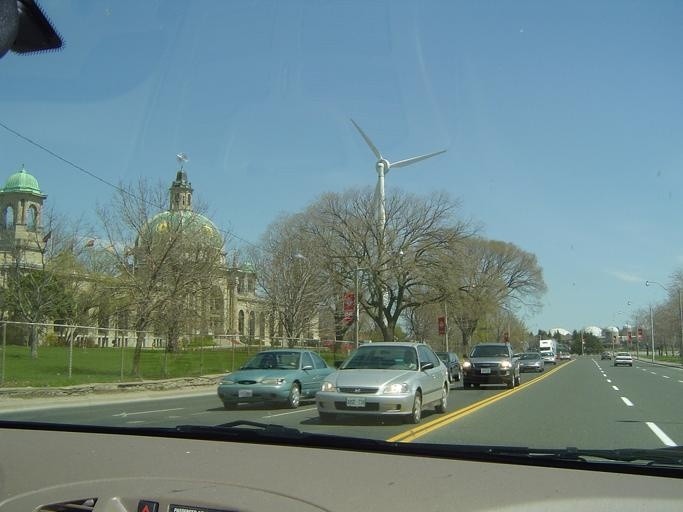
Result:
[355,247,404,347]
[627,298,655,363]
[644,280,682,360]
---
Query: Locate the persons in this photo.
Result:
[389,350,418,370]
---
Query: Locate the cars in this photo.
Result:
[317,339,450,425]
[613,353,633,368]
[601,350,612,361]
[433,349,462,385]
[463,338,571,386]
[217,348,339,410]
